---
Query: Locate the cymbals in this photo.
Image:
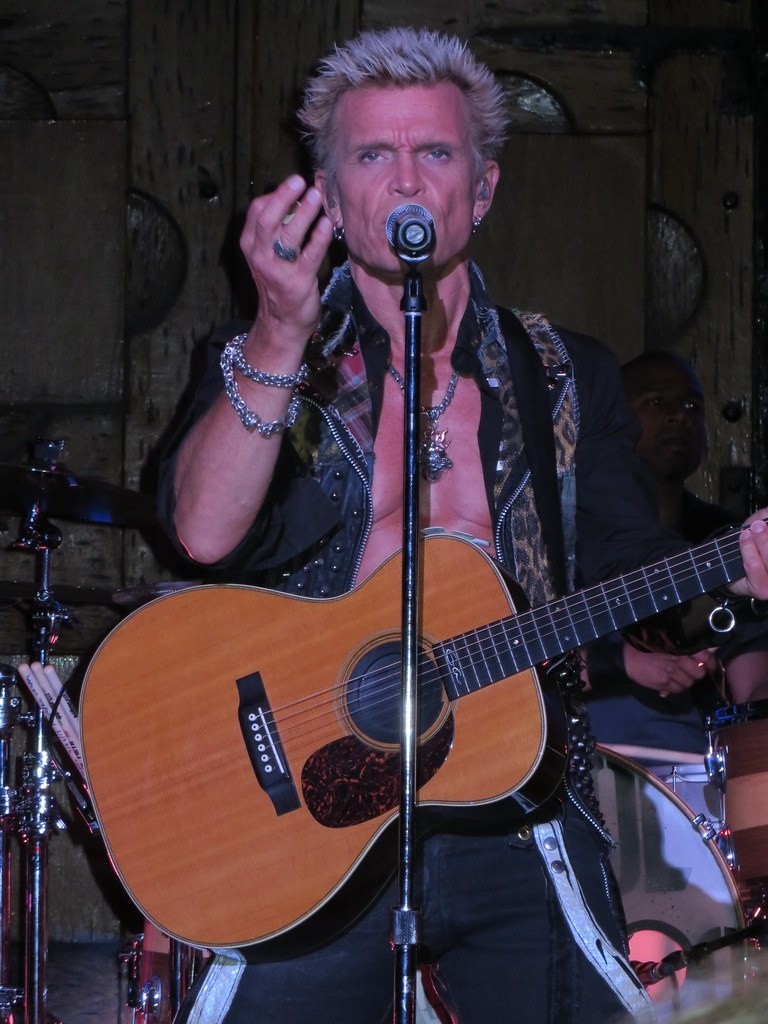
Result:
[1,458,154,530]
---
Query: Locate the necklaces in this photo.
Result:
[387,365,458,482]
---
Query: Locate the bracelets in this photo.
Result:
[221,333,309,435]
[586,640,628,690]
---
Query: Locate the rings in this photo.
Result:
[272,240,298,262]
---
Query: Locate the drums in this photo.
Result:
[608,741,725,846]
[709,695,766,942]
[584,738,748,1024]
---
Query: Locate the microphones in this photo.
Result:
[385,204,436,252]
[629,961,666,989]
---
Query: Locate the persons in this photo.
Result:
[136,24,768,1022]
[579,350,767,705]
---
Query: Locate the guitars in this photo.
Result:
[89,519,768,962]
